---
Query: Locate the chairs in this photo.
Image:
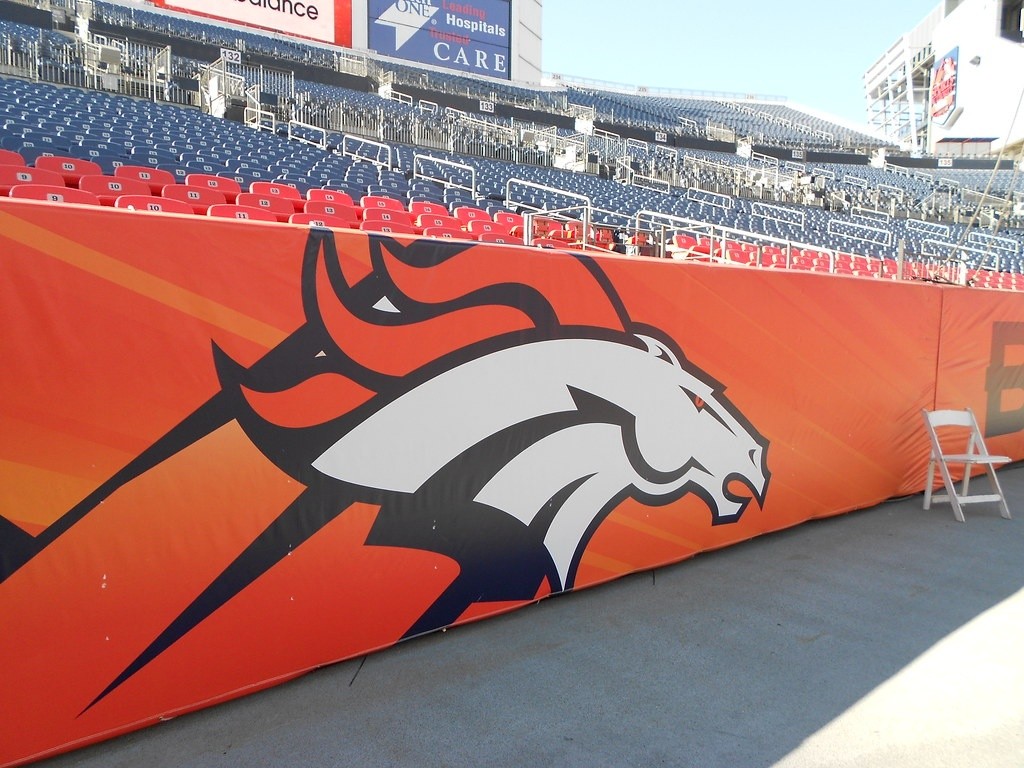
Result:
[922,408,1012,523]
[0,0,1024,292]
